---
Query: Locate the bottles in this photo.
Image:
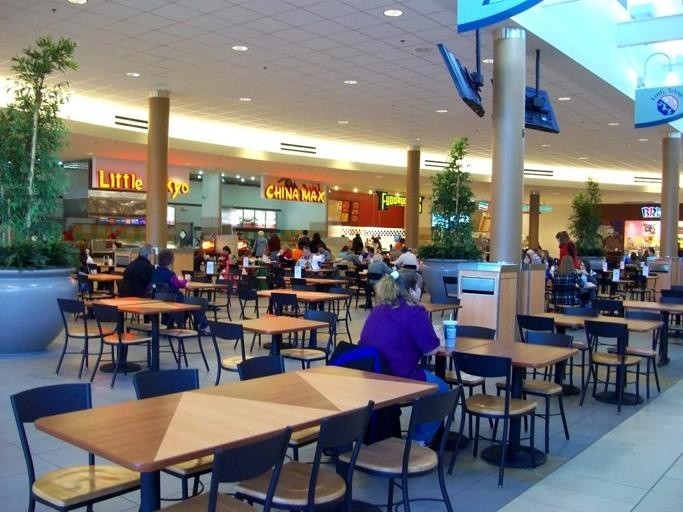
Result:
[389,244,393,250]
[102,256,108,265]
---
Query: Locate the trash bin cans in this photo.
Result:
[648,258,671,290]
[113,247,138,294]
[457,261,520,342]
[514,263,545,342]
[167,248,194,277]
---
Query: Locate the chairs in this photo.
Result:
[10,251,683,512]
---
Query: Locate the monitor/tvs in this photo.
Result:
[438,43,485,118]
[525,86,559,133]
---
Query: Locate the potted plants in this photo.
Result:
[0,33,77,356]
[569,178,607,270]
[416,137,483,305]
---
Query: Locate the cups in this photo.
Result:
[107,259,113,265]
[250,259,254,265]
[442,320,457,339]
[263,254,269,262]
[441,339,456,353]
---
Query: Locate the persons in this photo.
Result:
[628,247,655,280]
[395,247,416,266]
[298,230,327,253]
[576,260,597,300]
[556,231,579,269]
[278,244,330,270]
[339,234,382,267]
[601,228,624,270]
[195,246,232,269]
[96,242,121,291]
[123,245,211,336]
[358,270,452,448]
[524,248,553,277]
[368,253,393,283]
[678,243,683,257]
[75,240,89,292]
[395,238,405,250]
[252,230,281,258]
[553,255,585,305]
